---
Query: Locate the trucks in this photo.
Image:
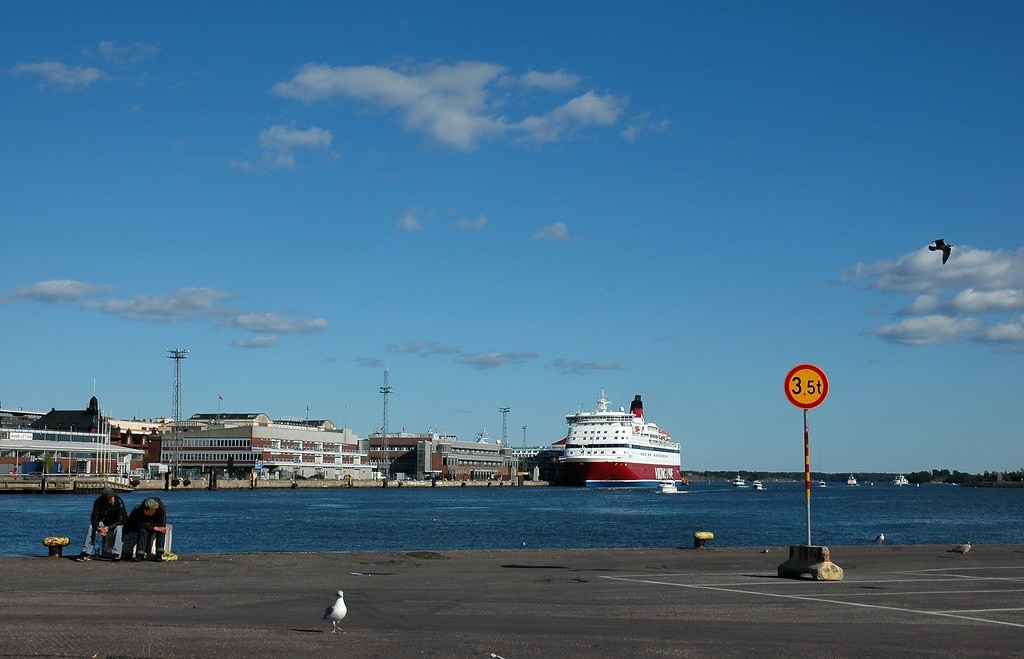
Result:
[372,472,386,480]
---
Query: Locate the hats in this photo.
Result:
[145,499,159,509]
[103,488,114,500]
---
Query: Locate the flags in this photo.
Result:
[219,395,223,400]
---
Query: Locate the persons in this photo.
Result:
[183,477,191,486]
[125,498,166,562]
[171,476,180,487]
[73,487,129,561]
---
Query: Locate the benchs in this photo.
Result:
[97,524,173,556]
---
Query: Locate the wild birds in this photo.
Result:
[323,590,347,634]
[945,540,972,558]
[928,238,955,266]
[872,533,885,546]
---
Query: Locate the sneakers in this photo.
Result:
[76,551,91,561]
[130,554,144,562]
[155,553,162,562]
[112,553,122,562]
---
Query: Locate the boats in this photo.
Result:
[656,474,678,493]
[537,385,685,489]
[733,475,745,486]
[846,475,857,485]
[681,477,691,486]
[752,480,767,490]
[819,480,826,488]
[893,476,908,486]
